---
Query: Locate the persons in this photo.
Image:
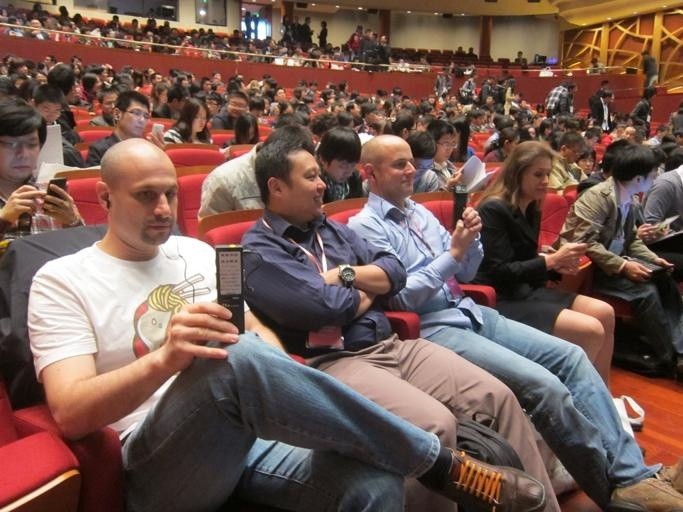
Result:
[348,132,682,511]
[239,134,568,511]
[26,136,548,511]
[1,0,683,388]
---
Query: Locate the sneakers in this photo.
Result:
[607,456,683,510]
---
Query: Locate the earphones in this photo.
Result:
[106,199,111,209]
[371,172,376,177]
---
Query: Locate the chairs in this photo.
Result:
[529,194,571,291]
[321,195,497,341]
[561,184,648,320]
[415,191,496,309]
[196,201,421,367]
[0,224,186,511]
[0,6,682,238]
[1,391,80,509]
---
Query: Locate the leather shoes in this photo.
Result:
[431,447,547,512]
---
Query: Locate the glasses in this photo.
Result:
[125,110,150,119]
[414,162,435,171]
[2,140,42,152]
[437,141,457,148]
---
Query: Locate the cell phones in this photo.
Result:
[214,245,244,335]
[43,178,67,213]
[454,184,468,229]
[152,124,164,141]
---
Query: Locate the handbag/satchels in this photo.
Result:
[454,409,526,472]
[614,353,663,376]
[547,394,645,495]
